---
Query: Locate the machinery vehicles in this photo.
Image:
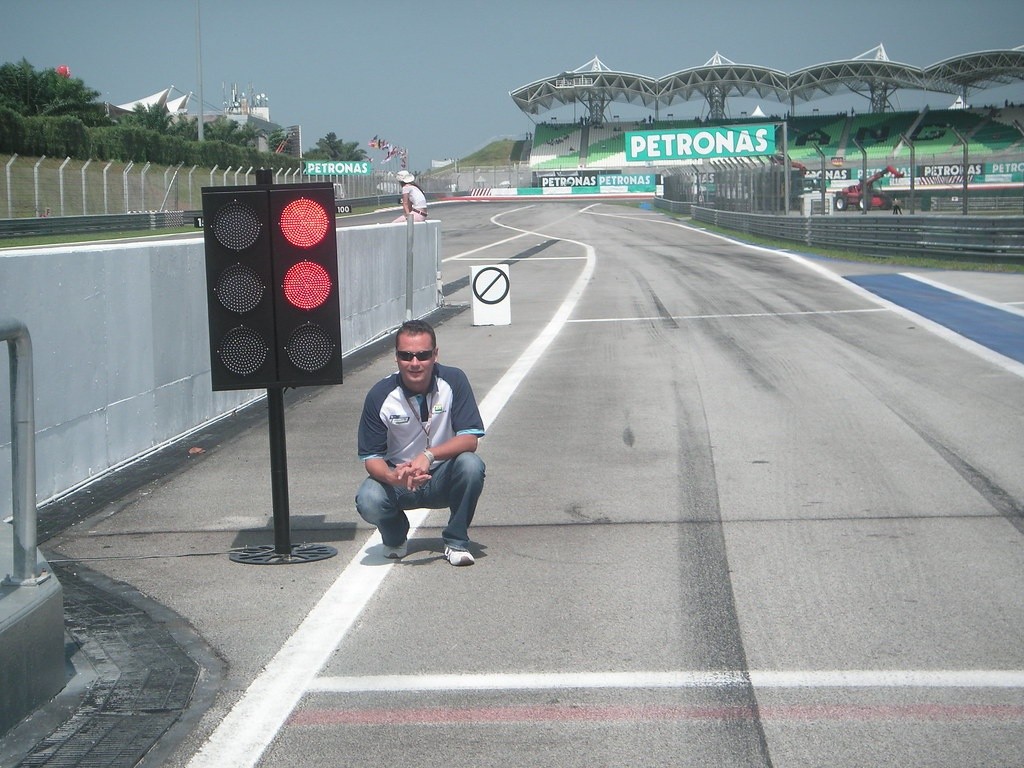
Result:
[834,165,905,211]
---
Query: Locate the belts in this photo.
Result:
[412,208,428,217]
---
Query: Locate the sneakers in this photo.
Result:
[382,535,407,558]
[444,542,475,565]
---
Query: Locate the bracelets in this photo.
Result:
[420,451,436,463]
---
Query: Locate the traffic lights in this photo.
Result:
[201,185,278,392]
[268,180,343,386]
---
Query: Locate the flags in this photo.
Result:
[367,135,408,167]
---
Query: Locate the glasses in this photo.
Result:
[396,346,435,361]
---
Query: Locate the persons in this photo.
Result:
[356,321,486,566]
[389,170,425,227]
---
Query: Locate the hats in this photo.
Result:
[396,170,415,183]
